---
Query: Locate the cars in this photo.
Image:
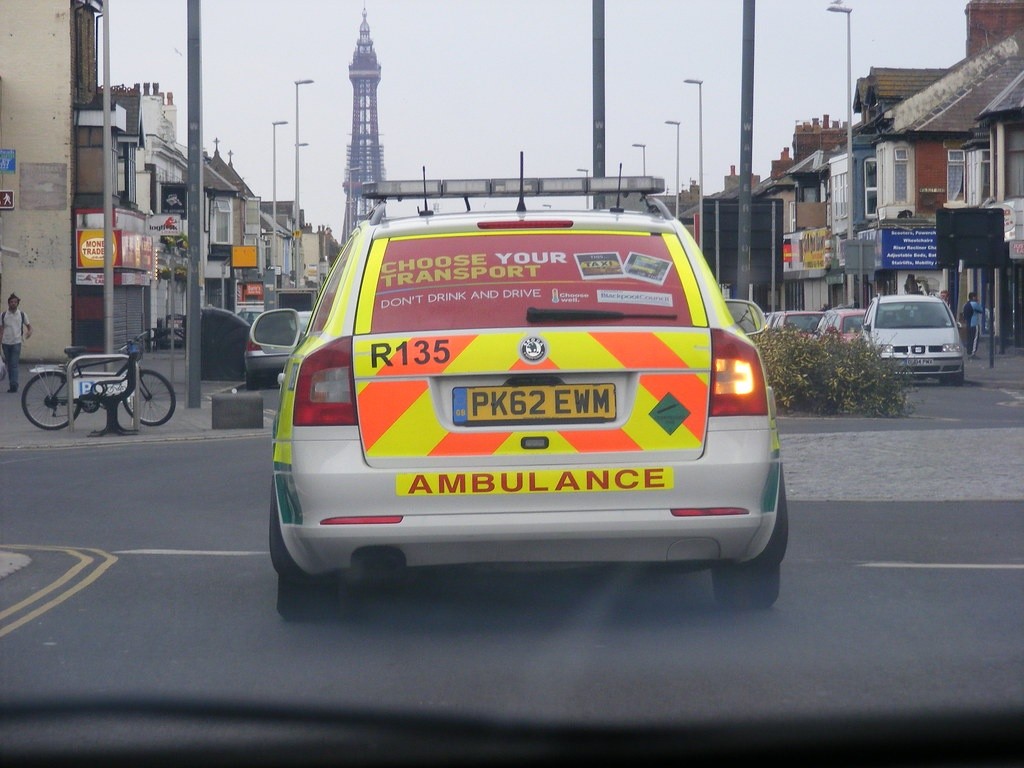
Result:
[862,294,971,381]
[247,310,315,389]
[767,308,865,342]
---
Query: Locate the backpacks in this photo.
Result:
[964,302,974,320]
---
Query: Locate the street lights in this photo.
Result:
[631,142,646,177]
[271,120,287,285]
[683,77,704,255]
[294,79,315,270]
[826,4,854,312]
[666,119,679,220]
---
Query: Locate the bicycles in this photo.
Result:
[23,333,180,429]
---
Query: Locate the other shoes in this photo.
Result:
[7,389,17,393]
[968,355,981,360]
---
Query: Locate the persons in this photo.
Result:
[0,291,33,393]
[961,292,984,360]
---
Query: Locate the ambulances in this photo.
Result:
[245,162,791,621]
